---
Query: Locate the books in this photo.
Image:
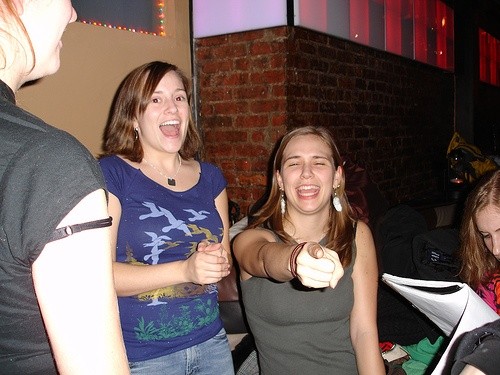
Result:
[383,272,500,375]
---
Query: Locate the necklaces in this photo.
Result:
[140,150,183,186]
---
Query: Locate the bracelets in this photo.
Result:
[288,242,305,278]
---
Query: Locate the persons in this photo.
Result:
[229,124,387,375]
[0,0,139,375]
[448,319,499,375]
[375,164,500,347]
[96,61,234,375]
